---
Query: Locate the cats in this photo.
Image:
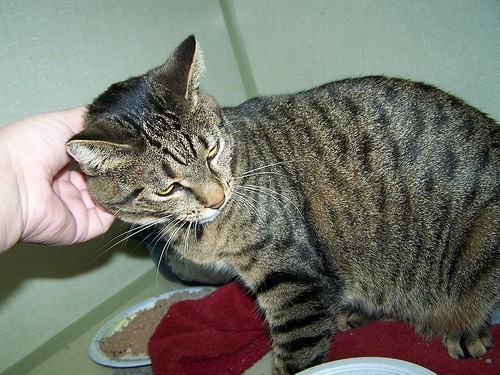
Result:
[64,34,499,375]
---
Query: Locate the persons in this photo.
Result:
[0,105,118,260]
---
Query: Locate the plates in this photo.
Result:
[294,356,435,375]
[89,286,216,366]
